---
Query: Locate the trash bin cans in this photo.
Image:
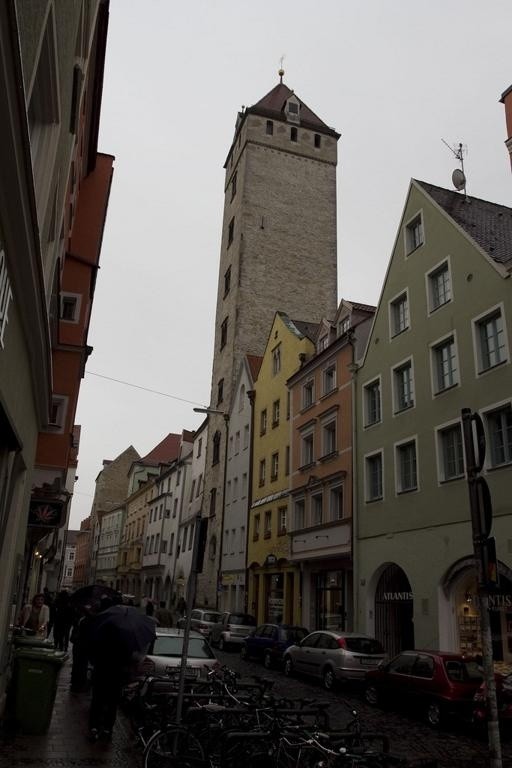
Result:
[8,639,70,735]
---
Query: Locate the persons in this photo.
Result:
[17,582,187,740]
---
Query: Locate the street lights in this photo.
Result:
[192,406,229,609]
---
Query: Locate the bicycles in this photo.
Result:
[131,701,404,768]
